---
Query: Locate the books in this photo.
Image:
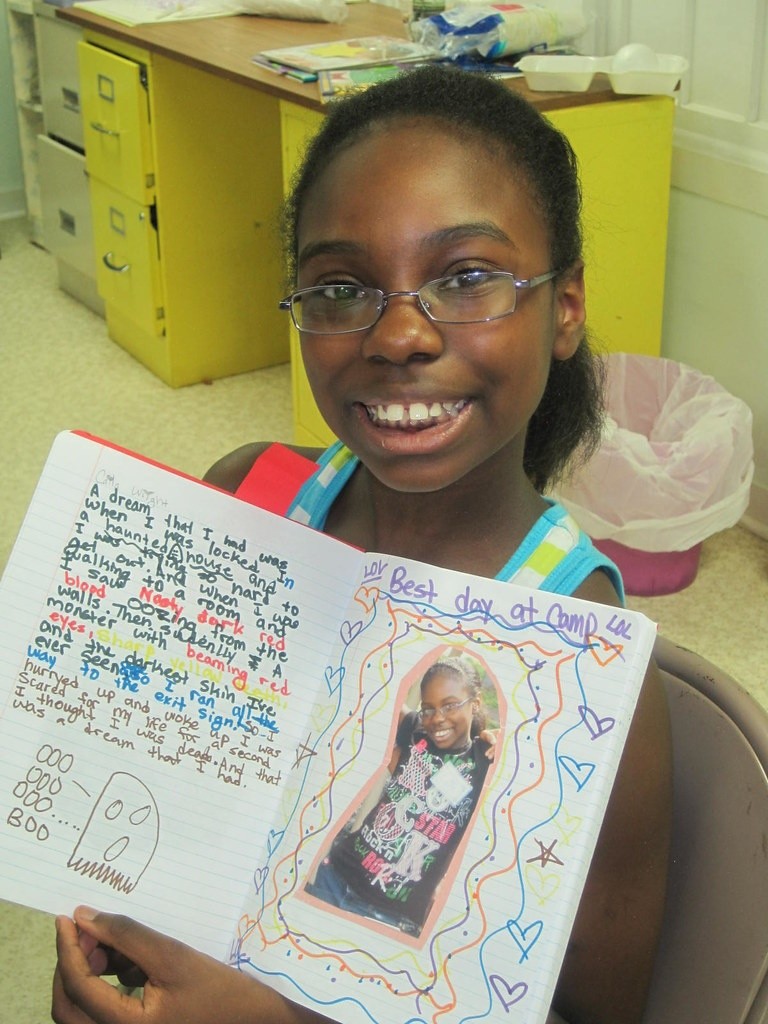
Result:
[250,31,439,107]
[0,422,662,1024]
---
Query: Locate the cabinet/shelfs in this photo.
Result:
[5,1,684,455]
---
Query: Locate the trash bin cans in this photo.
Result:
[557,349,732,597]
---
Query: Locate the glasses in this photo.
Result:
[414,694,474,720]
[279,253,568,335]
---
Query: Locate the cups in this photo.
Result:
[440,2,588,59]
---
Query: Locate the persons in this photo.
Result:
[310,653,503,934]
[46,58,672,1023]
